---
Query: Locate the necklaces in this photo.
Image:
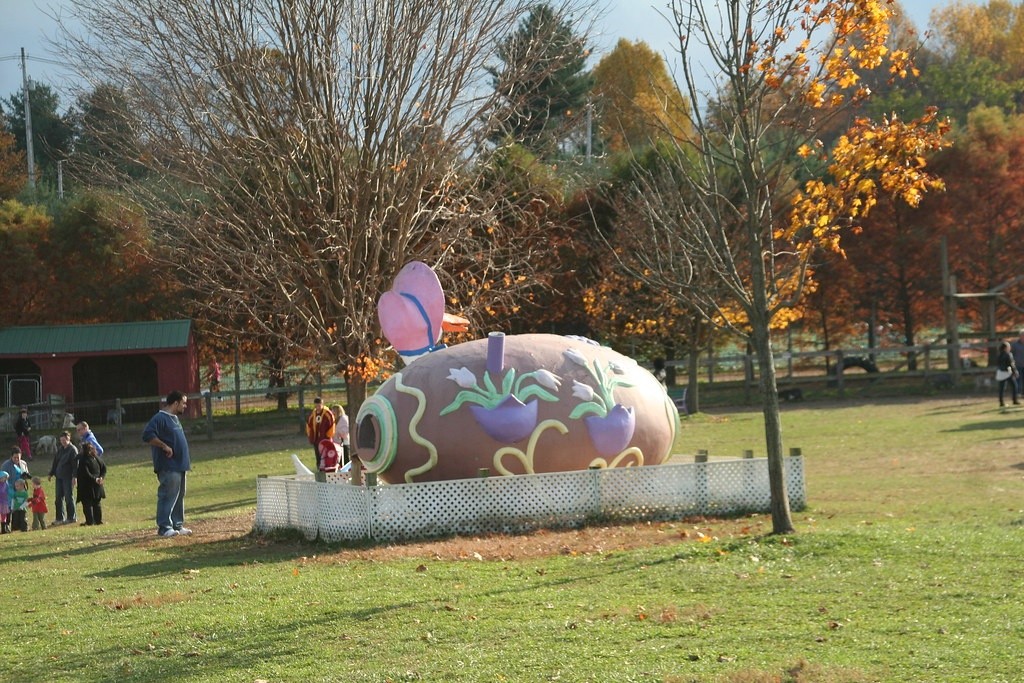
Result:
[165,409,179,425]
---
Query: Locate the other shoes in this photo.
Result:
[64,518,77,524]
[80,521,92,527]
[96,520,103,525]
[52,517,64,525]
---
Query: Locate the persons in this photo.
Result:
[651,356,668,398]
[996,329,1024,408]
[142,390,193,538]
[306,397,335,471]
[332,404,350,468]
[0,407,106,534]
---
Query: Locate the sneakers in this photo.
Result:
[164,528,179,537]
[177,527,192,536]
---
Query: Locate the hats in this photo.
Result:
[0,470,9,480]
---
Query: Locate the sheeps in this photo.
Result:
[33,435,58,455]
[106,407,125,424]
[61,412,77,427]
